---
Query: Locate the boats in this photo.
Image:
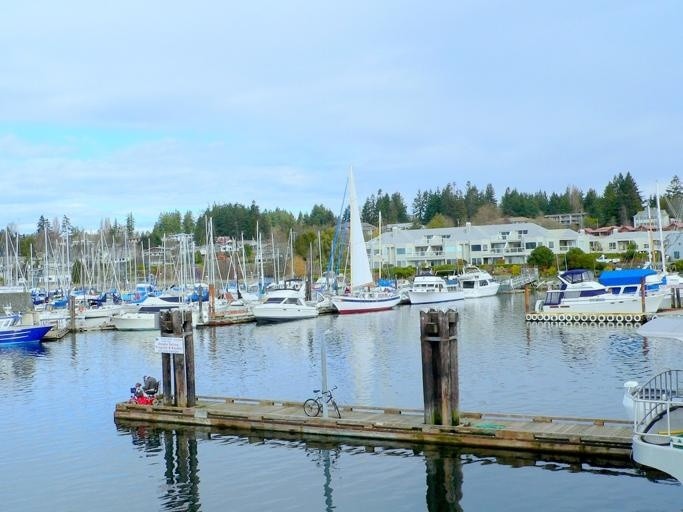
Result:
[533,180,683,314]
[620,368,683,490]
[109,296,199,331]
[455,270,499,300]
[2,324,57,348]
[249,289,319,326]
[406,273,465,304]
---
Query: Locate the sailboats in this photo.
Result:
[3,167,460,324]
[333,166,404,313]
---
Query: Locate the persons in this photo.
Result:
[141,376,157,394]
[133,382,152,405]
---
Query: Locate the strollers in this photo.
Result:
[128,375,160,406]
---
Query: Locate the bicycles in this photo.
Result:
[303,384,342,420]
